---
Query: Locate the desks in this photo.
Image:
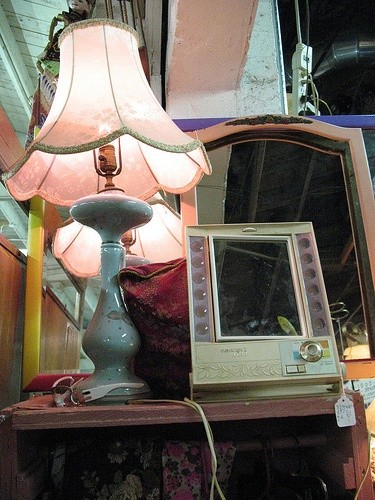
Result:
[0,394,372,500]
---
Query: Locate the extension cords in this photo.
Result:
[292,43,313,117]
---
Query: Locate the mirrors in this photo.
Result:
[182,114,375,380]
[23,124,198,392]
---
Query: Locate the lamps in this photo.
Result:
[54,199,181,274]
[3,18,212,405]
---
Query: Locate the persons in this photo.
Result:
[41,0,96,63]
